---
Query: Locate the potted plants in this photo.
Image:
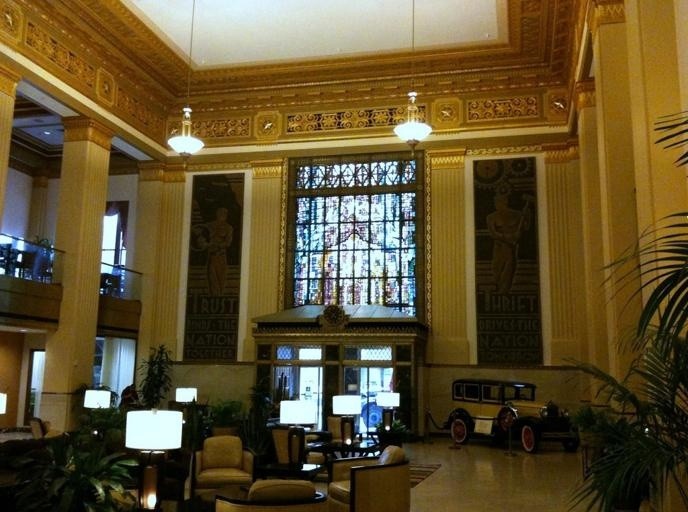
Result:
[212,400,242,436]
[570,405,606,460]
[376,420,407,451]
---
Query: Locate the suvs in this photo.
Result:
[360,388,382,428]
[449,379,579,455]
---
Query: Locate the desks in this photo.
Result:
[321,439,380,468]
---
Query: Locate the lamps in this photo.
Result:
[84,389,111,409]
[393,1,432,157]
[168,0,205,170]
[376,392,400,431]
[332,395,362,445]
[175,387,197,421]
[279,400,317,470]
[125,408,183,511]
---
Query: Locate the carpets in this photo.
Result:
[409,461,443,489]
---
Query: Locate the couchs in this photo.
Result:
[327,416,362,443]
[215,479,327,512]
[266,422,328,479]
[327,446,410,511]
[191,435,256,501]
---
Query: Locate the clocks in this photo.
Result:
[321,305,345,327]
